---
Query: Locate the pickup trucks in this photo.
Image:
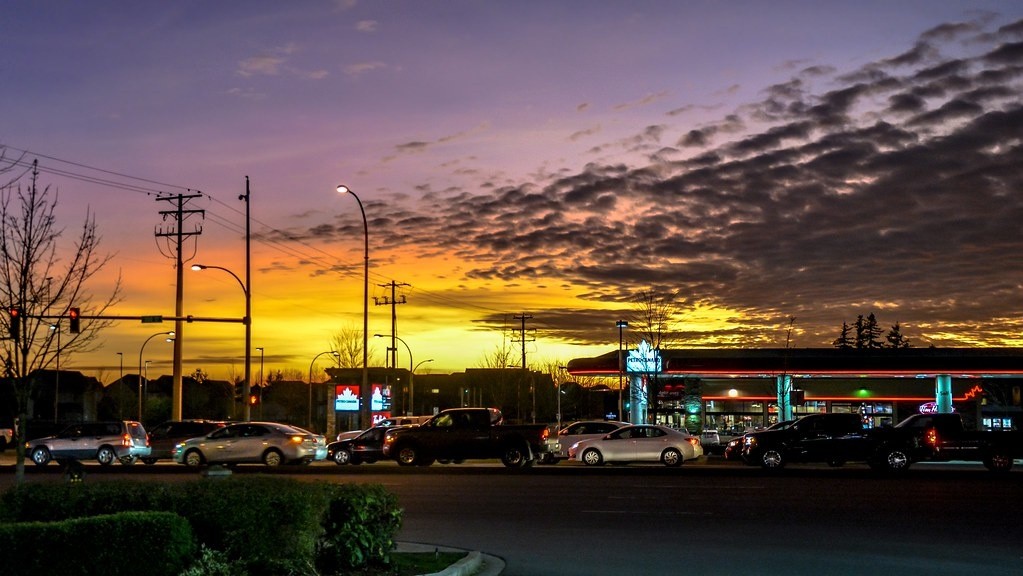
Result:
[25,421,152,466]
[867,413,1023,472]
[384,408,561,468]
[337,416,434,442]
[0,429,13,452]
[742,413,872,466]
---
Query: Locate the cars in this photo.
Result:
[724,419,796,465]
[171,421,316,467]
[717,425,745,449]
[310,432,328,463]
[568,425,704,466]
[558,421,632,458]
[327,426,411,465]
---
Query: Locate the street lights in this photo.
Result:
[50,323,61,434]
[374,334,414,416]
[309,351,338,431]
[616,320,628,423]
[409,359,433,416]
[192,264,252,421]
[336,185,369,430]
[117,353,123,378]
[386,346,398,382]
[256,348,264,421]
[145,360,152,400]
[139,331,175,422]
[558,366,567,432]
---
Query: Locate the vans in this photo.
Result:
[141,420,235,465]
[674,428,720,449]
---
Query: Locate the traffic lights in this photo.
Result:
[69,307,80,333]
[12,309,20,339]
